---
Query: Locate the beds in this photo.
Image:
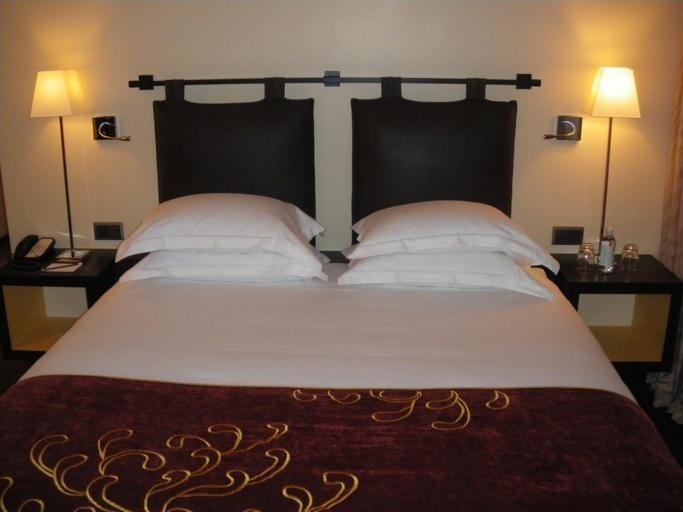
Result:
[1,263,683,512]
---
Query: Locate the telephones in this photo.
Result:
[14,234,54,265]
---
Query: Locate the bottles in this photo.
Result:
[619,243,639,273]
[576,242,595,275]
[598,227,616,273]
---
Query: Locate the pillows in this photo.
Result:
[340,200,561,275]
[337,252,556,302]
[115,192,326,264]
[119,249,329,283]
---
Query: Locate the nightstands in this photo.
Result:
[1,249,121,360]
[549,253,682,373]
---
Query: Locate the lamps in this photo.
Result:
[583,66,642,274]
[30,69,93,264]
